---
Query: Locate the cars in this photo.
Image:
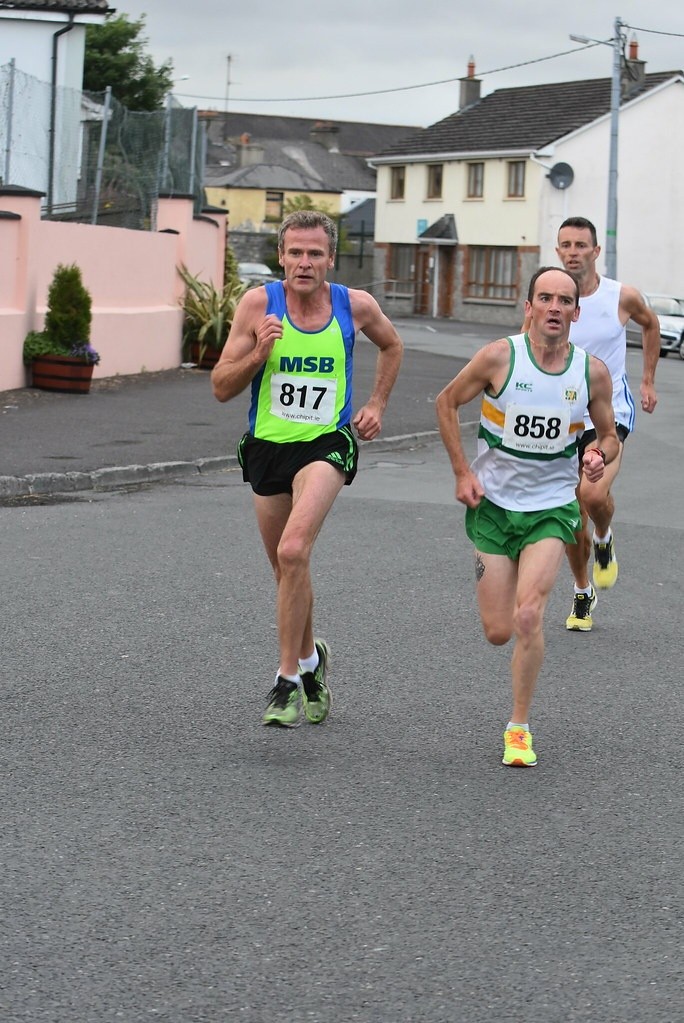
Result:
[627,290,684,362]
[237,261,281,288]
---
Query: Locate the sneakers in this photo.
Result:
[567,587,598,630]
[262,675,304,723]
[501,725,537,766]
[296,638,333,722]
[591,528,618,589]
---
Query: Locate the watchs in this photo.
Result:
[590,447,607,462]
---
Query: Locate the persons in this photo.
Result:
[520,217,661,632]
[435,265,619,768]
[211,206,402,728]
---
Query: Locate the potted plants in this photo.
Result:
[22,262,100,399]
[172,259,233,370]
